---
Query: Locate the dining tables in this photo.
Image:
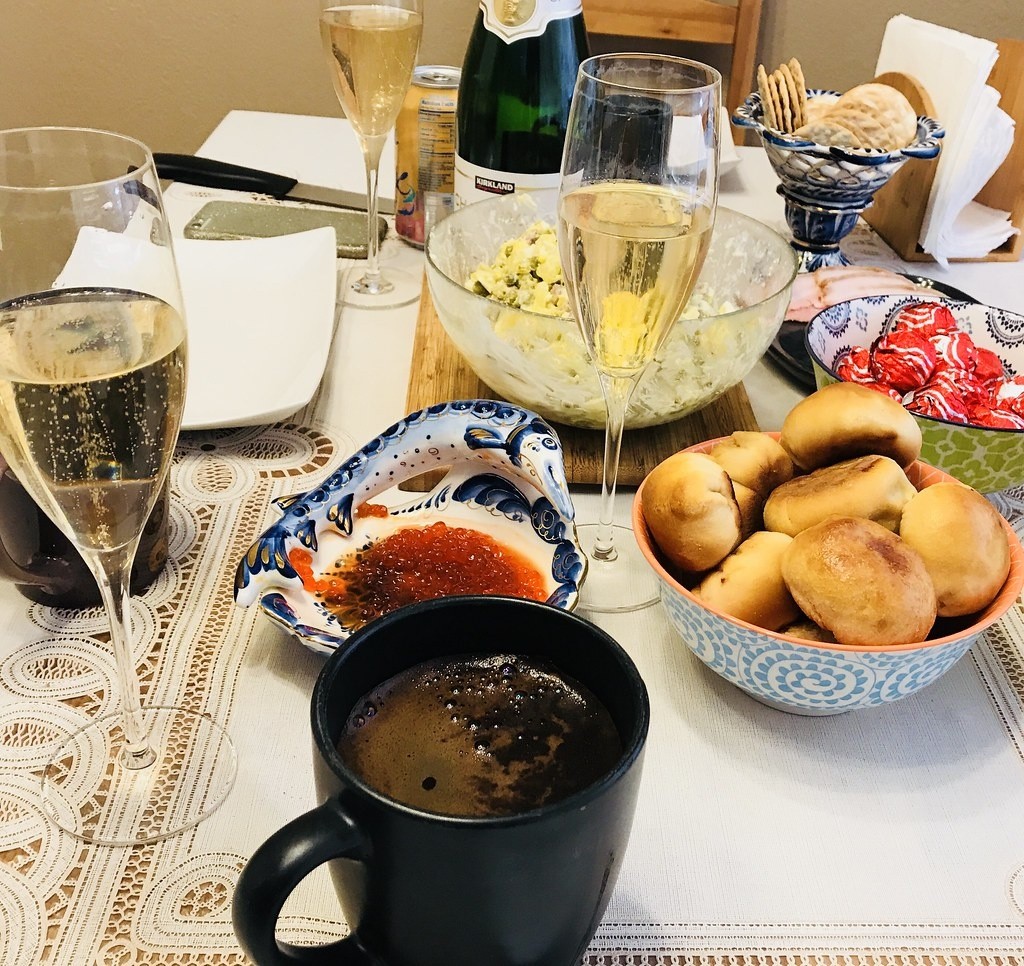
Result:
[0,106,1024,966]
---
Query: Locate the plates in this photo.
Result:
[52,219,339,432]
[671,109,742,181]
[762,268,985,398]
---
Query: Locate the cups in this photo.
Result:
[0,451,169,609]
[231,594,653,966]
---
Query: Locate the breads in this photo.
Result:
[640,381,1013,647]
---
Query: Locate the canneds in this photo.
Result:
[393,64,466,250]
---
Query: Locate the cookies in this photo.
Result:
[756,56,917,151]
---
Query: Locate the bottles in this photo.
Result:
[450,0,592,212]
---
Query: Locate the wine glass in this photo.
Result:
[725,80,946,270]
[318,0,423,307]
[0,126,242,847]
[554,51,723,613]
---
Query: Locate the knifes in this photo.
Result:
[151,148,397,215]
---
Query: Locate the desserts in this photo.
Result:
[838,302,1024,430]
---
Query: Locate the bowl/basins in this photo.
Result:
[631,430,1023,719]
[423,187,798,430]
[805,291,1024,493]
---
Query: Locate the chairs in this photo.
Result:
[581,1,764,144]
